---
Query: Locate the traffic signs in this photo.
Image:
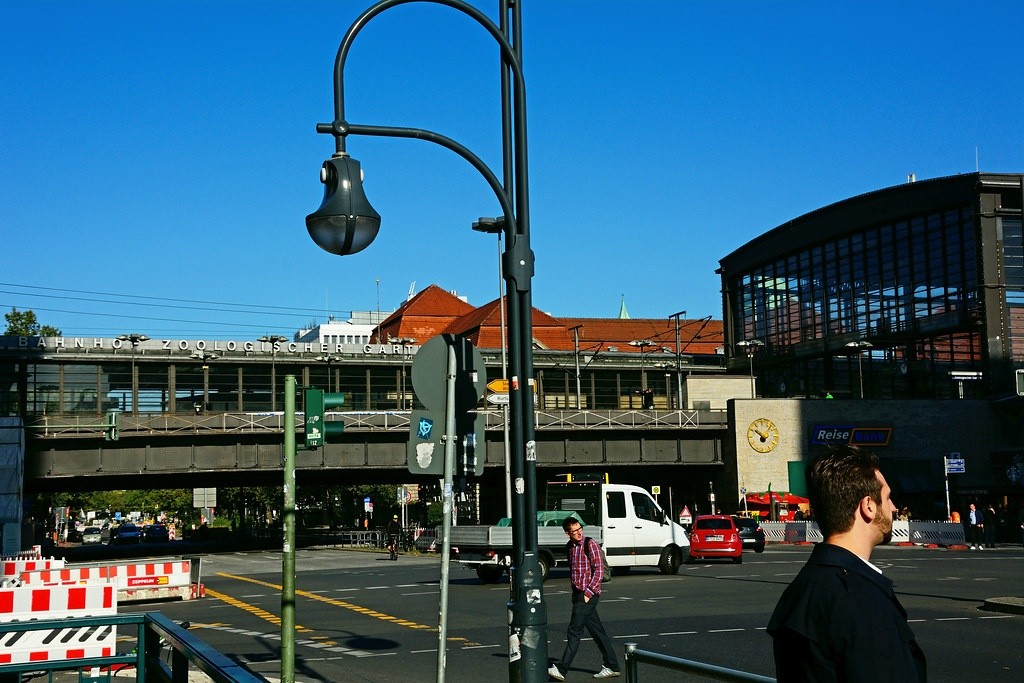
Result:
[486,393,537,405]
[485,379,537,393]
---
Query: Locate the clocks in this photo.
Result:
[747,418,779,453]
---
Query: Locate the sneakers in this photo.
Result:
[547,664,564,681]
[593,665,620,678]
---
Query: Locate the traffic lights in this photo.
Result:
[304,384,346,448]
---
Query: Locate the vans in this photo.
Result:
[115,523,139,545]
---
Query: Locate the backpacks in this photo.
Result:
[584,537,612,583]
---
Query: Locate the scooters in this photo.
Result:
[385,532,405,561]
[402,530,415,552]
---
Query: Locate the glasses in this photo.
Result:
[568,526,583,534]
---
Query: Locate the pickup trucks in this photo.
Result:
[434,471,691,586]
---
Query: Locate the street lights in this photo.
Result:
[257,333,289,412]
[628,338,657,409]
[845,340,874,400]
[737,340,765,399]
[305,0,550,683]
[115,333,151,417]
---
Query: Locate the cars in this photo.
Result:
[687,515,743,562]
[143,524,170,543]
[135,525,153,542]
[731,517,766,553]
[81,527,103,546]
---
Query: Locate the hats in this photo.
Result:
[393,515,399,518]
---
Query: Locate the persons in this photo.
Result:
[639,386,653,410]
[548,517,620,681]
[273,517,281,528]
[386,515,403,552]
[766,445,928,683]
[230,517,238,532]
[181,519,207,540]
[892,502,1024,550]
[707,509,753,519]
[791,508,816,521]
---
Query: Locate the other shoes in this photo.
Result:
[970,546,975,550]
[978,546,983,550]
[991,544,995,548]
[985,544,989,548]
[397,554,399,557]
[388,546,390,550]
